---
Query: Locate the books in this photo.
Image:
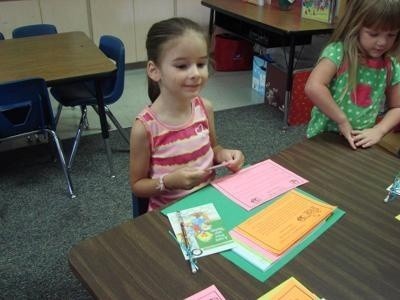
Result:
[302,0,340,25]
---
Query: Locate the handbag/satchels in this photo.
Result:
[212,33,260,72]
[251,56,268,95]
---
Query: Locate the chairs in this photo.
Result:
[0,23,129,200]
[133,181,147,216]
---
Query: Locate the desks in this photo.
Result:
[200,0,337,134]
[69,123,400,300]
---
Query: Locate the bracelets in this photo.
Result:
[160,173,173,192]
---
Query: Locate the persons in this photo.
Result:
[304,0,400,150]
[128,17,245,214]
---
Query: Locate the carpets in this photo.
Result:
[0,103,400,300]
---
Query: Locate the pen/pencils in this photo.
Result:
[177,210,197,273]
[384,176,400,203]
[207,162,231,170]
